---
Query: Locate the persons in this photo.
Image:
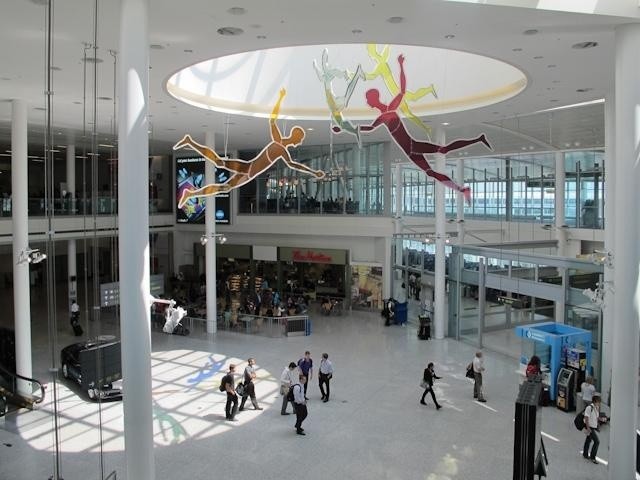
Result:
[420,363,444,410]
[473,350,486,402]
[150,259,423,330]
[525,355,545,407]
[586,395,607,464]
[298,351,313,401]
[239,358,263,410]
[364,44,440,140]
[581,375,595,409]
[332,52,493,200]
[219,365,238,420]
[313,49,368,150]
[318,351,334,402]
[279,362,298,415]
[68,298,80,326]
[172,85,325,208]
[291,374,307,435]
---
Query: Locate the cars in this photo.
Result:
[61,338,123,402]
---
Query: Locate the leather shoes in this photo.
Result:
[295,425,304,431]
[323,398,329,402]
[281,413,290,415]
[321,394,326,399]
[297,432,306,435]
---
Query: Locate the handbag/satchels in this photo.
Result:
[218,373,229,391]
[235,383,247,396]
[419,380,430,391]
[574,405,593,431]
[465,361,474,379]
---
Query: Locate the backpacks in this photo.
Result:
[287,384,301,401]
